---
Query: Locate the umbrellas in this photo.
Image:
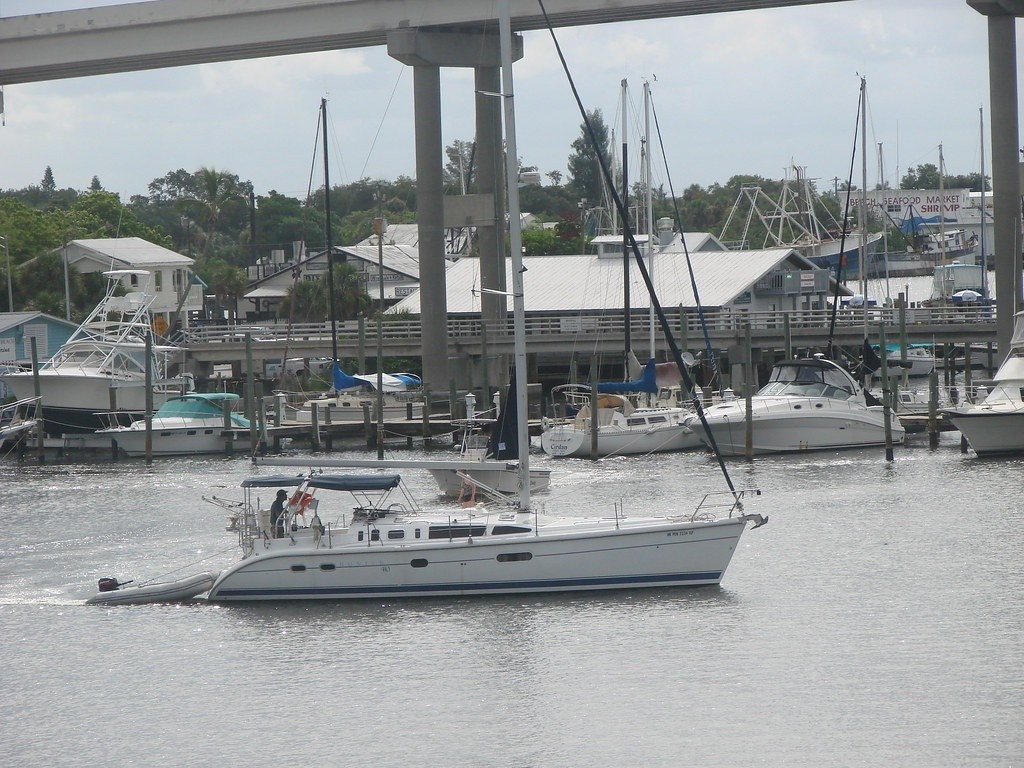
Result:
[951,288,983,314]
[849,295,877,310]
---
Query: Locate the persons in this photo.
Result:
[269,489,290,539]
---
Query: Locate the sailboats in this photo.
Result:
[78,13,769,611]
[0,61,1024,453]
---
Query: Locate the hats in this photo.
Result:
[277,490,288,496]
